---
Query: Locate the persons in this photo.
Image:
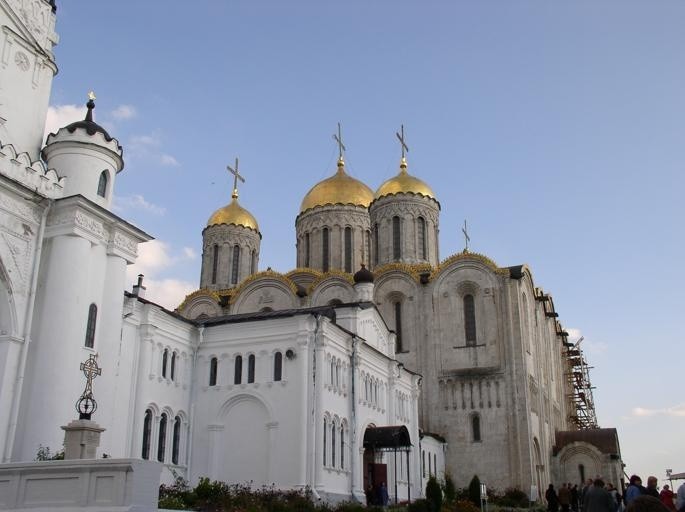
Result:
[545,473,684,510]
